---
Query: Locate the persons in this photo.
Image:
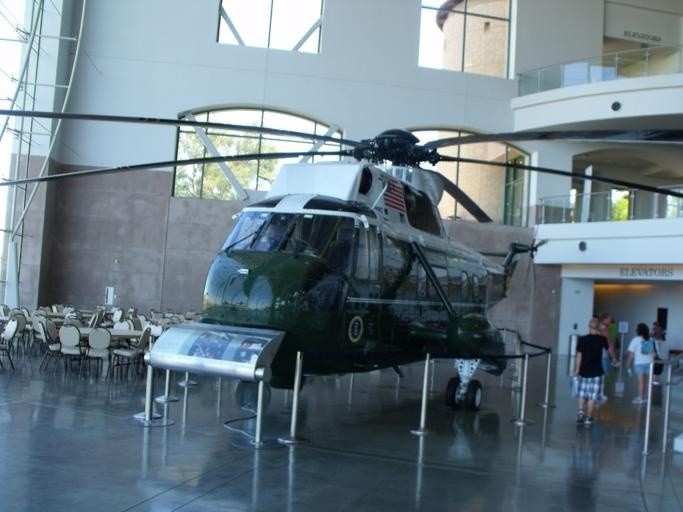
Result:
[596,313,620,401]
[625,323,658,405]
[607,319,617,345]
[649,320,658,337]
[574,316,609,426]
[379,174,406,214]
[565,424,603,512]
[628,404,653,478]
[265,220,288,253]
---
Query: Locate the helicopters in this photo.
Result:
[0,105,683,412]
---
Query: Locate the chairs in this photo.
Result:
[0,302,211,385]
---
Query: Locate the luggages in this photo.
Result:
[653,375,662,406]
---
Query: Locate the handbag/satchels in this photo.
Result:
[662,333,666,340]
[615,338,620,349]
[654,356,663,375]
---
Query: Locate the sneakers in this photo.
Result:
[632,396,647,404]
[578,409,593,425]
[597,392,608,401]
